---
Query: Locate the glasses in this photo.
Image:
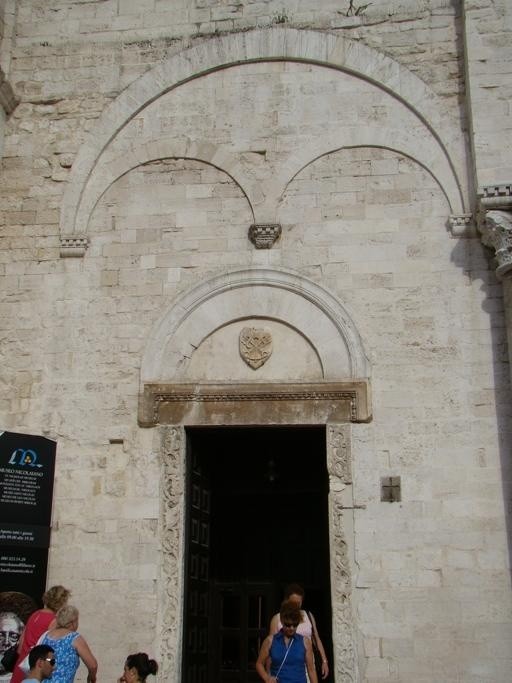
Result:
[283,621,299,627]
[40,657,56,666]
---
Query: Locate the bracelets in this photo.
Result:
[321,660,329,664]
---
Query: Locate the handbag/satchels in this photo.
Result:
[311,633,320,656]
[1,645,17,672]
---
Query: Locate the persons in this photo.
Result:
[117,652,159,682]
[28,606,98,683]
[22,644,57,683]
[11,584,71,683]
[1,612,26,683]
[263,590,328,682]
[256,601,319,682]
